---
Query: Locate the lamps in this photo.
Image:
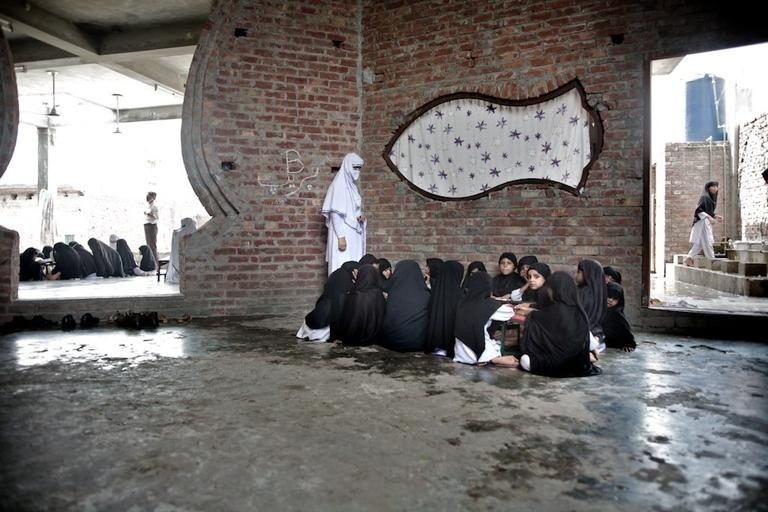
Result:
[35,71,61,116]
[111,93,123,134]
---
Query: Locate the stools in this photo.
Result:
[157,255,171,281]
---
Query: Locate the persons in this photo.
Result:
[295,251,636,376]
[143,191,161,257]
[321,153,368,278]
[19,233,160,280]
[683,182,723,266]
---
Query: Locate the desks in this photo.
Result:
[489,299,539,355]
[38,260,56,278]
